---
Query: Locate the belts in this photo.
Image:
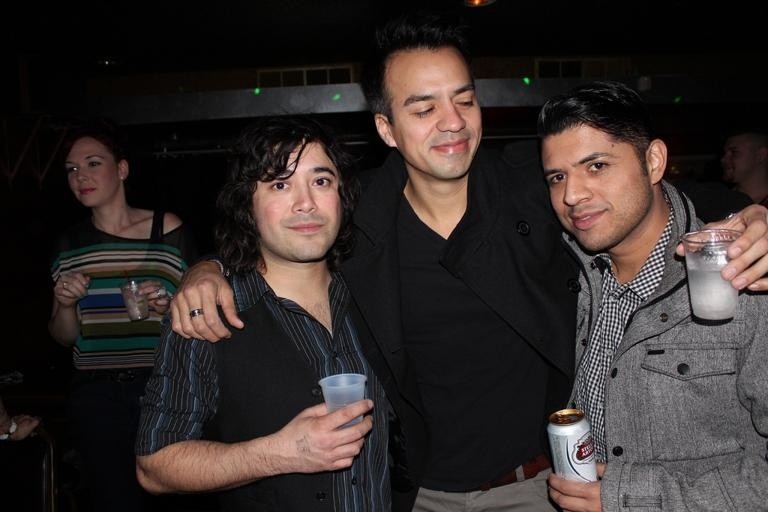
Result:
[494,451,553,487]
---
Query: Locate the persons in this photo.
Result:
[721,133,768,208]
[48,130,215,512]
[539,82,768,512]
[135,113,407,512]
[0,397,40,442]
[169,14,768,512]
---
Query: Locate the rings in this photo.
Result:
[157,289,162,298]
[190,308,203,317]
[63,282,68,289]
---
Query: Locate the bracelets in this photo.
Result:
[208,259,224,274]
[0,422,18,440]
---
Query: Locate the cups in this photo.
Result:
[121,282,149,323]
[682,229,739,322]
[319,374,368,426]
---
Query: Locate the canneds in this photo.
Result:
[547,407,599,483]
